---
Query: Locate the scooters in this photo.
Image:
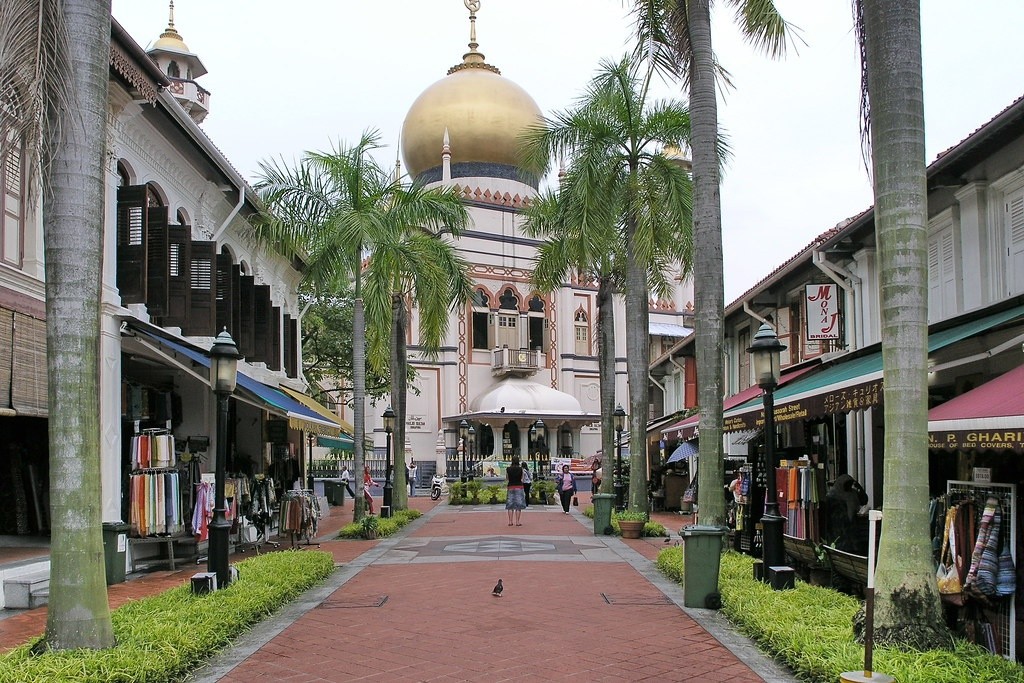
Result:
[429,472,447,501]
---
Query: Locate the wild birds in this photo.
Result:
[491,578,504,595]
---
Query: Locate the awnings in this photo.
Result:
[928,364,1024,452]
[137,327,354,450]
[649,322,690,337]
[659,305,1024,441]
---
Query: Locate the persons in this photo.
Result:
[556,465,578,515]
[521,462,532,503]
[364,465,379,514]
[341,466,355,499]
[505,455,526,526]
[405,463,417,496]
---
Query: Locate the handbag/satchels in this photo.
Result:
[574,495,578,506]
[934,497,1018,607]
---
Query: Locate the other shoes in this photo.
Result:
[369,511,375,514]
[516,523,522,526]
[508,523,514,526]
[563,510,569,516]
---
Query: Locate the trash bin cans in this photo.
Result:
[678,525,730,610]
[591,493,617,534]
[320,480,336,503]
[332,482,348,506]
[102,521,132,585]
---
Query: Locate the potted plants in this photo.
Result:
[449,479,558,505]
[616,509,650,538]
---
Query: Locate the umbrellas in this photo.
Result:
[666,442,699,463]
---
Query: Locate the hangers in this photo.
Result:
[280,490,314,501]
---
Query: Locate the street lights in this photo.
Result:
[530,426,538,482]
[535,418,548,505]
[307,432,317,496]
[467,425,475,482]
[613,402,628,515]
[189,326,245,596]
[459,419,470,499]
[745,319,797,591]
[380,404,398,518]
